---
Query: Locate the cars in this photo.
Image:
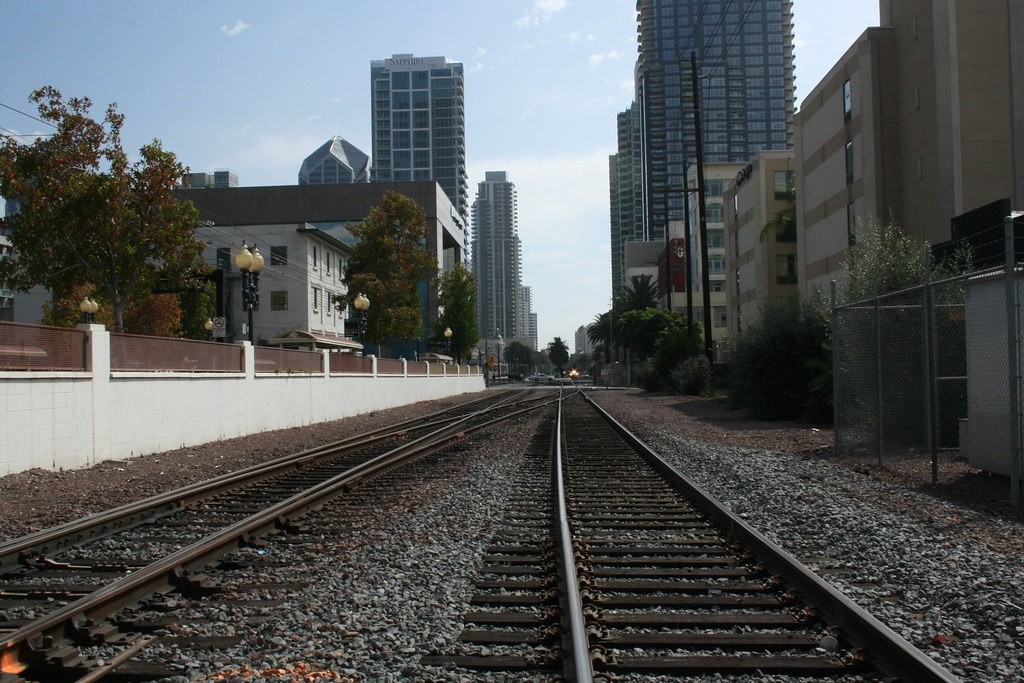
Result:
[531,374,553,382]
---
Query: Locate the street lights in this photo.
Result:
[354,293,370,355]
[236,239,264,345]
[444,327,452,357]
[80,295,99,370]
[205,317,213,340]
